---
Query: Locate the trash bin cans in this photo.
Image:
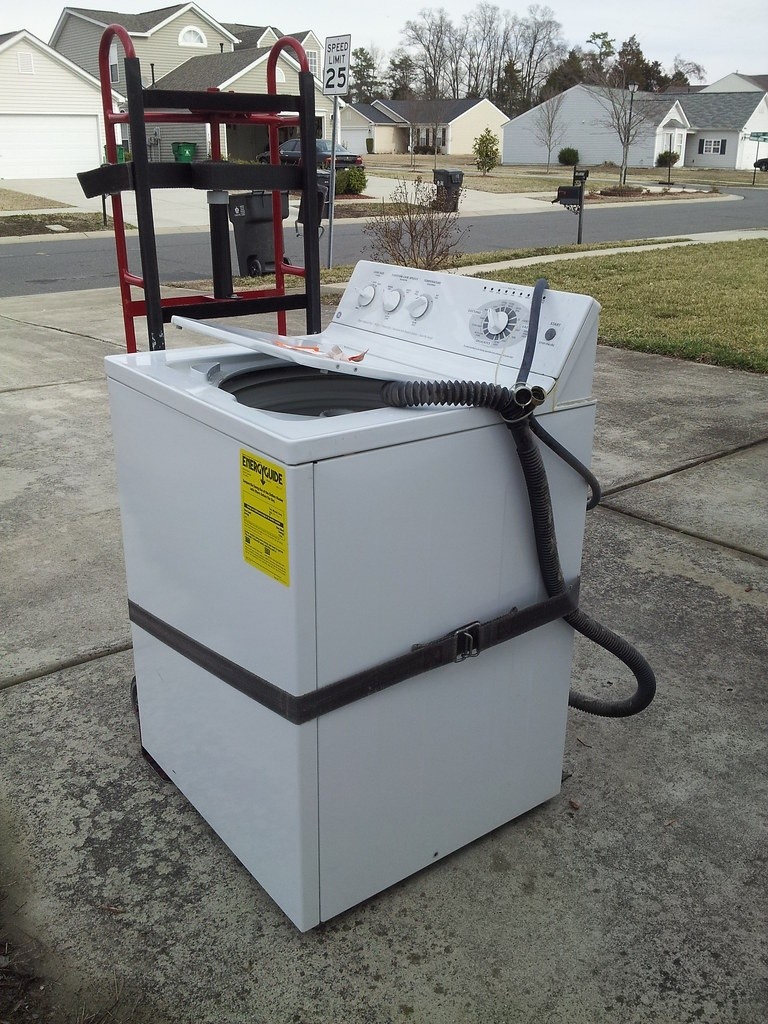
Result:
[103,144,125,164]
[431,167,464,213]
[227,188,293,277]
[171,142,197,163]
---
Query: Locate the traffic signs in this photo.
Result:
[748,132,768,143]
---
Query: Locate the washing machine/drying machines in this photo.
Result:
[106,258,605,936]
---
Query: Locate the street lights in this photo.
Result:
[623,80,640,185]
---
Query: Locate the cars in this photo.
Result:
[412,145,442,156]
[753,158,768,172]
[255,137,365,179]
